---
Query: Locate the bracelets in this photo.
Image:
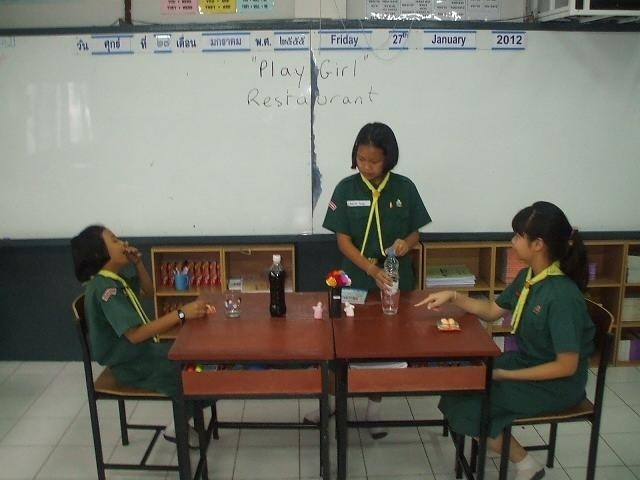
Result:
[177,308,186,324]
[450,289,462,306]
[366,262,374,277]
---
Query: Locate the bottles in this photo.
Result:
[267,255,288,316]
[384,249,400,297]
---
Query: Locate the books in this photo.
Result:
[339,287,368,305]
[469,247,604,354]
[228,276,243,290]
[618,251,640,362]
[426,263,476,288]
[241,276,292,293]
[348,361,409,369]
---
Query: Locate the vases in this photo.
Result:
[330,287,341,317]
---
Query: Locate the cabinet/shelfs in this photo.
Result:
[615,239,639,367]
[422,238,627,370]
[151,242,298,342]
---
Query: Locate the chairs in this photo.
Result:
[73,292,213,480]
[469,296,616,480]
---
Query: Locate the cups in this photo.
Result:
[380,288,400,314]
[174,274,189,291]
[222,290,242,319]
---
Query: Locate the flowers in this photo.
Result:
[325,269,352,288]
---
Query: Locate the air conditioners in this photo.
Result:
[532,1,640,24]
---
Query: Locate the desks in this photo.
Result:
[329,290,502,480]
[169,288,335,479]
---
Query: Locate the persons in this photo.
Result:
[302,120,434,439]
[70,222,213,450]
[414,201,601,479]
